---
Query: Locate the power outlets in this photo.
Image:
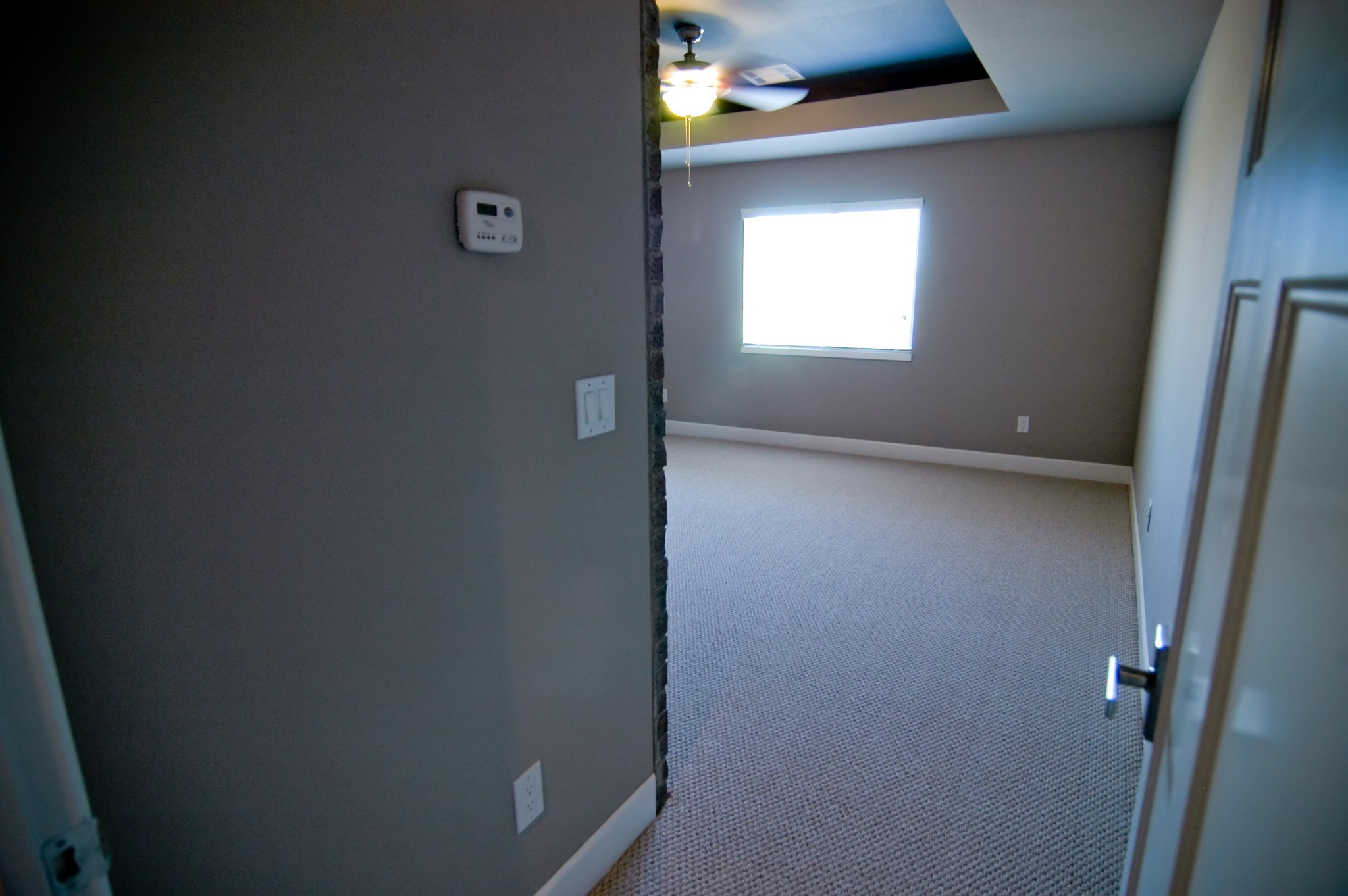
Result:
[1017,416,1029,433]
[513,761,544,834]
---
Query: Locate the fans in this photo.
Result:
[660,26,809,112]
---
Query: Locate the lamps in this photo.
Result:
[663,88,717,186]
